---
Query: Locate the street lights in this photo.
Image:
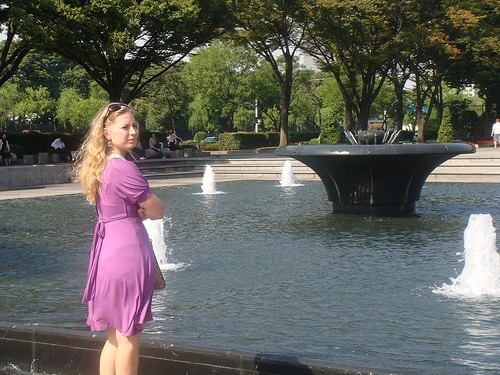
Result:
[206,106,211,136]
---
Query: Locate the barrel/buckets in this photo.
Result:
[38,153,48,163]
[184,148,192,158]
[52,154,59,163]
[23,155,34,164]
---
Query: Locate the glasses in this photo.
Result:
[104,103,131,123]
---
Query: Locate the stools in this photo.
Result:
[53,153,59,162]
[38,153,49,164]
[184,148,192,157]
[23,155,34,165]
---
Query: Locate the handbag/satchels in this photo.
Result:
[149,241,165,289]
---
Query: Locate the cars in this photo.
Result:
[204,137,218,143]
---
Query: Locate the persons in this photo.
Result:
[491,119,500,149]
[0,133,13,166]
[168,131,183,159]
[51,136,75,164]
[75,105,166,375]
[318,133,323,144]
[131,135,148,160]
[149,132,167,159]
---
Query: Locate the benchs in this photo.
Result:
[148,148,171,158]
[0,153,16,164]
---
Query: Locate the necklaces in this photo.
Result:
[114,152,126,160]
